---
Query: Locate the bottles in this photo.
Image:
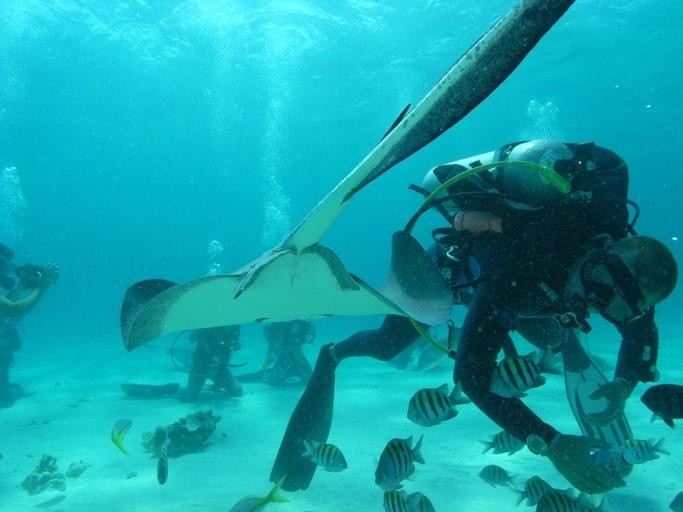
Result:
[591,448,638,479]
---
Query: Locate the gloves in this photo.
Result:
[544,433,626,495]
[584,378,632,426]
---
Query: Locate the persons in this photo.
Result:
[0,243,59,406]
[256,320,316,387]
[317,236,677,495]
[157,320,243,403]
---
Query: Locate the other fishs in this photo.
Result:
[157,438,172,485]
[302,439,347,472]
[477,344,683,512]
[374,433,425,492]
[383,491,409,512]
[120,0,578,353]
[406,380,472,427]
[227,473,291,512]
[406,491,436,512]
[111,418,133,456]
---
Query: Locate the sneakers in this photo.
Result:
[269,343,340,492]
[121,383,178,397]
[235,371,264,381]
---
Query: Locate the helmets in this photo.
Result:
[614,284,646,323]
[592,236,678,302]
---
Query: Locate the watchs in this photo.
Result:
[526,422,555,457]
